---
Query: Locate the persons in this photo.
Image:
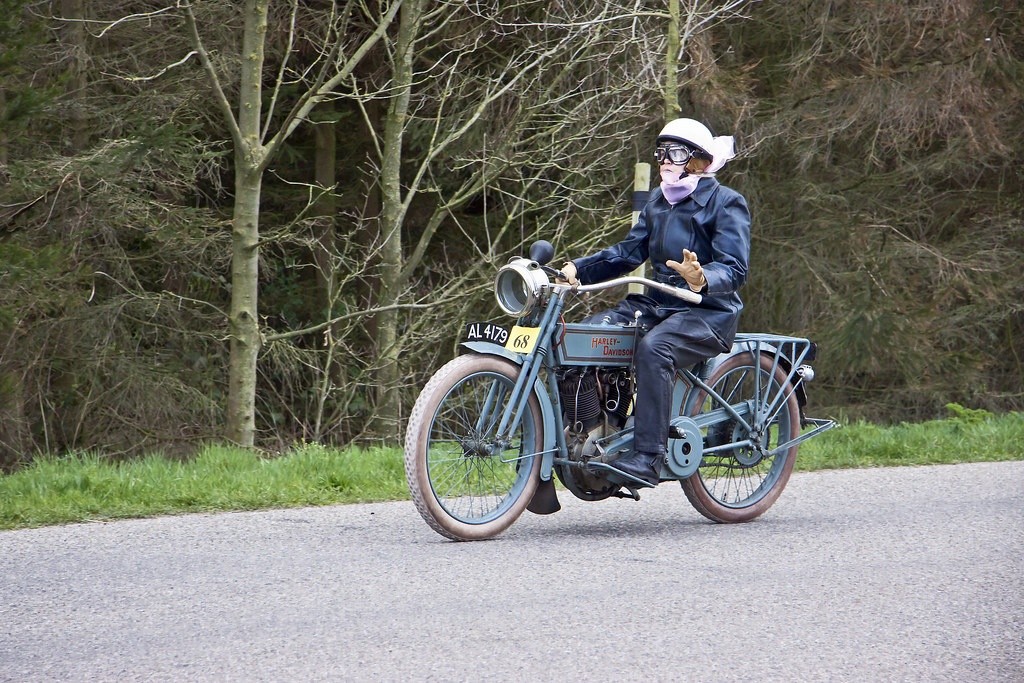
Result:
[553,118,751,487]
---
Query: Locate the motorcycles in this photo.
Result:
[402,241,844,544]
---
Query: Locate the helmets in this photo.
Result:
[656,117,714,164]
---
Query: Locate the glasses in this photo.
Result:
[653,144,712,165]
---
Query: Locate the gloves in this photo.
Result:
[554,262,577,285]
[666,248,707,293]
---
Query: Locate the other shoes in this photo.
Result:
[606,450,661,488]
